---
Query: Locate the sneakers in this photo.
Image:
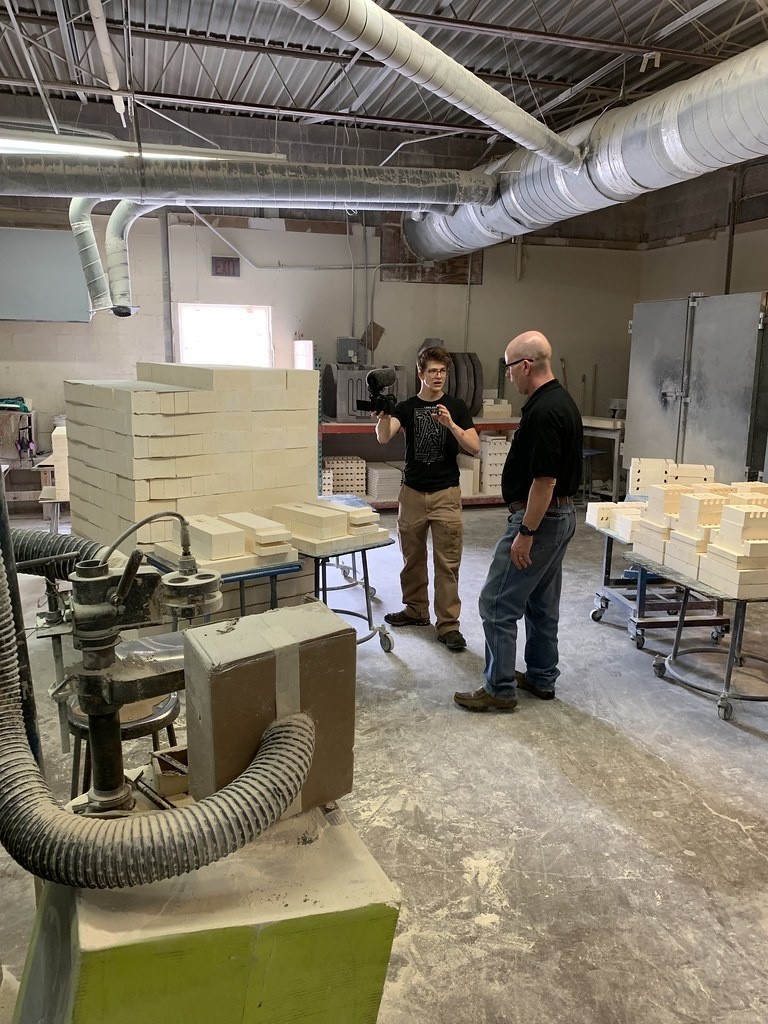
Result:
[384,610,431,626]
[515,672,556,700]
[454,685,518,711]
[437,630,467,649]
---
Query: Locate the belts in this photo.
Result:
[508,496,571,512]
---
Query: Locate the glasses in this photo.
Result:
[425,369,445,377]
[504,358,533,373]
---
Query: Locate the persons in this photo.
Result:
[454,331,583,712]
[370,346,480,650]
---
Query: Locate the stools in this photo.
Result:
[65,691,180,801]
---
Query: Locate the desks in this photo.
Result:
[581,429,624,504]
[144,550,304,626]
[30,461,70,534]
[299,535,395,652]
[584,521,768,720]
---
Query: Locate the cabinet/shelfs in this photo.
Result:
[314,344,521,509]
[621,289,768,483]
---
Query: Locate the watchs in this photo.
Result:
[519,525,536,536]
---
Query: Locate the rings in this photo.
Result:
[519,557,523,559]
